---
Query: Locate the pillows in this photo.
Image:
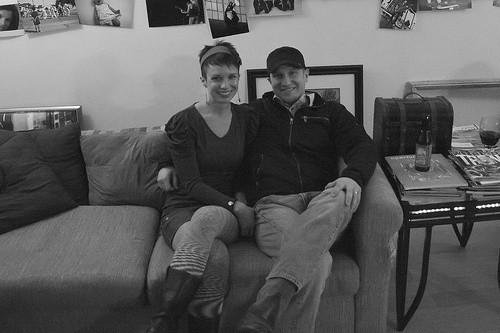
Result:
[80,130,166,210]
[0,122,89,236]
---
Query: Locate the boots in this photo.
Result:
[148,265,200,332]
[235,278,296,333]
[188,314,221,333]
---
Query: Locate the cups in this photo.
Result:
[479,116,500,147]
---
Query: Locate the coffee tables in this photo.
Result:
[382,164,500,330]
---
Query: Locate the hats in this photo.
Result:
[266,46,305,74]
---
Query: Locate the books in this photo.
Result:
[385,148,500,205]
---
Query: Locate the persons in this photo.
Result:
[0,5,20,31]
[94,0,121,26]
[153,40,257,333]
[31,6,41,32]
[158,45,380,333]
[20,2,70,20]
[181,0,200,24]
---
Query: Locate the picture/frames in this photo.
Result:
[405,78,500,138]
[0,105,82,132]
[247,65,363,125]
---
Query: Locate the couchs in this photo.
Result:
[0,125,403,333]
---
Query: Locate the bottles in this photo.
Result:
[415,113,431,171]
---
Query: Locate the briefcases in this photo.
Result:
[373,92,455,156]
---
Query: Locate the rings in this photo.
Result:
[353,191,358,194]
